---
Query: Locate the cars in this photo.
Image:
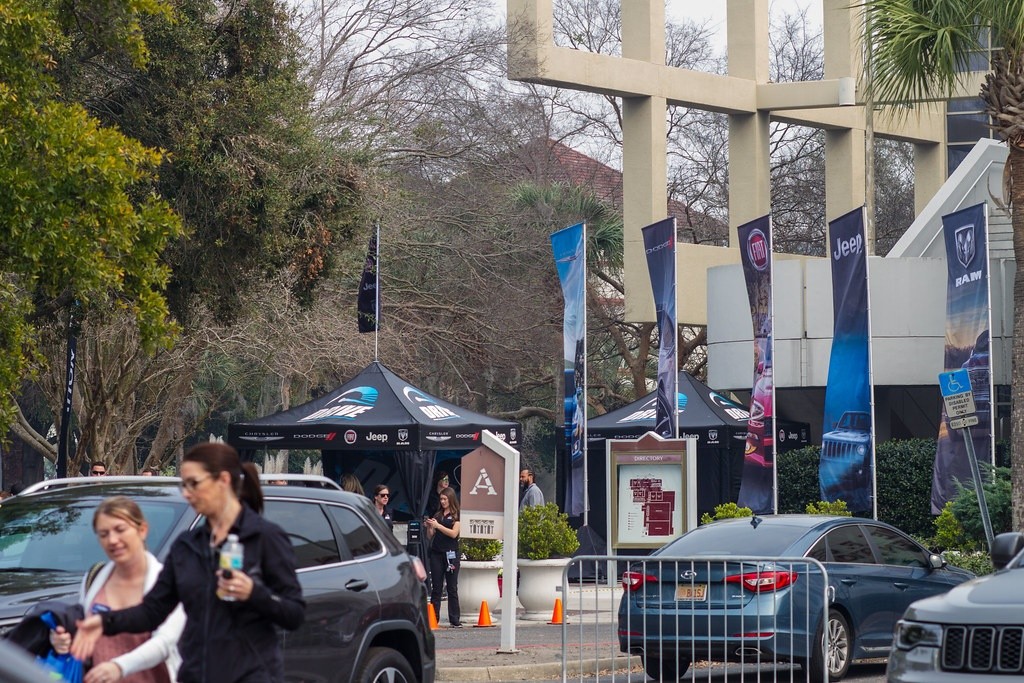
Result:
[745,332,774,468]
[617,509,978,680]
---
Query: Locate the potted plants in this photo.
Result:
[517,502,579,621]
[935,501,987,566]
[457,538,505,623]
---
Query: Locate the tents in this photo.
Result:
[227,360,521,596]
[555,370,811,558]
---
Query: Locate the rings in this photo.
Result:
[224,570,231,578]
[228,586,234,591]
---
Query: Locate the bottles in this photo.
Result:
[216,534,245,602]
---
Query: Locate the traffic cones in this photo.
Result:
[548,598,572,625]
[429,604,445,630]
[472,600,497,628]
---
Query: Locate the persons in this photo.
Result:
[142,468,157,476]
[70,443,307,683]
[91,461,106,476]
[373,485,393,534]
[338,472,364,494]
[268,481,288,485]
[0,483,25,502]
[51,497,185,683]
[422,471,463,628]
[519,470,546,520]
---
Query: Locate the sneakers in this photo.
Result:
[449,621,464,628]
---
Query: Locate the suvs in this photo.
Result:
[564,370,583,461]
[1,475,438,683]
[821,413,871,478]
[884,529,1024,683]
[946,328,991,436]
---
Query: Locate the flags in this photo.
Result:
[358,224,381,333]
[931,202,993,516]
[57,312,77,478]
[550,221,585,516]
[736,215,775,514]
[820,208,874,515]
[642,216,676,439]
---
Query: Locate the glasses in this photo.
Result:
[178,475,215,493]
[377,493,390,498]
[441,478,450,481]
[91,469,105,475]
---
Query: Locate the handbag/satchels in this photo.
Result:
[33,611,84,683]
